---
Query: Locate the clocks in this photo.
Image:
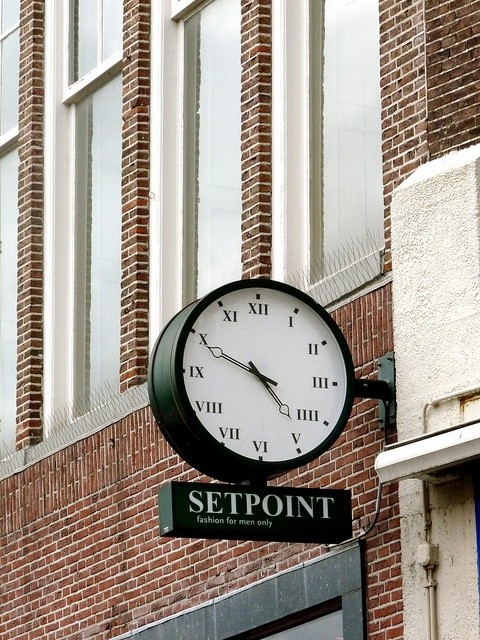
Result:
[148,279,357,483]
